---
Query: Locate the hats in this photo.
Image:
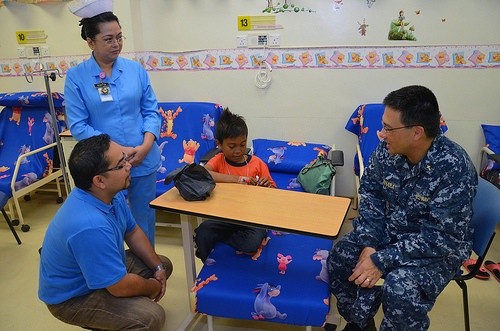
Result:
[73,0,113,18]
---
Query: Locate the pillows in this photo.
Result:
[253,138,331,174]
[0,90,65,108]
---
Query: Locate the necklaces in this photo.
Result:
[224,154,248,177]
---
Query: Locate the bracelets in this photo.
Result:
[153,264,165,271]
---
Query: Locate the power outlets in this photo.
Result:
[17,47,26,58]
[41,46,51,57]
[269,34,279,47]
[237,35,247,48]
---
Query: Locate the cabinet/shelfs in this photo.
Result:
[57,131,79,190]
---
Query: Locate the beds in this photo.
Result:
[195,140,343,331]
[480,123,500,189]
[344,102,450,210]
[0,92,71,246]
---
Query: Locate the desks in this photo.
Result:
[149,183,351,331]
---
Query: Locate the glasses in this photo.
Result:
[97,151,125,175]
[93,35,126,45]
[381,120,423,134]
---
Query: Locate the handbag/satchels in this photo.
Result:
[174,163,216,201]
[297,155,336,195]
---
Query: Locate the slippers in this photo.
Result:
[463,258,490,278]
[484,260,500,282]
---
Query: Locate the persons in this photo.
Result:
[195,113,276,264]
[38,133,173,331]
[63,0,161,250]
[327,85,479,331]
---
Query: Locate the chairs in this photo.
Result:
[452,176,500,331]
[148,101,222,236]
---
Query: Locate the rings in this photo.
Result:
[366,277,371,283]
